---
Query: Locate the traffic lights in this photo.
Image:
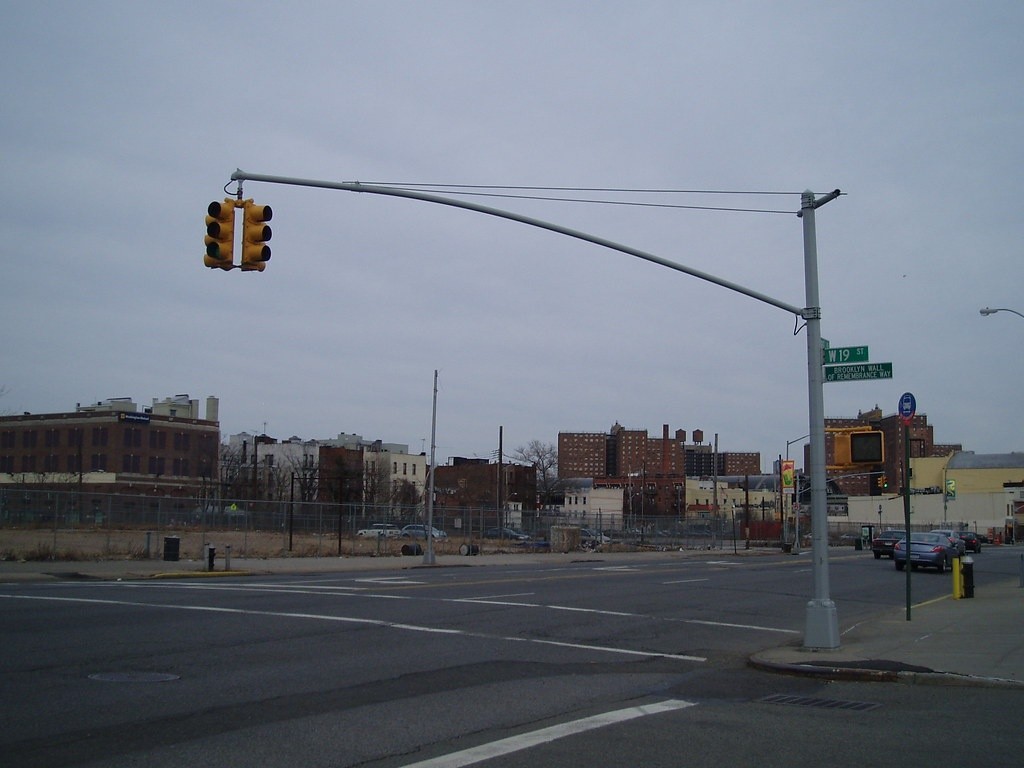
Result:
[883,477,888,488]
[878,477,883,487]
[849,430,884,464]
[204,200,235,270]
[242,202,271,271]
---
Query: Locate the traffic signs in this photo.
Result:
[824,346,869,364]
[826,361,893,381]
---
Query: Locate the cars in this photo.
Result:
[894,532,960,572]
[484,527,530,541]
[872,530,907,558]
[961,532,982,553]
[581,528,610,542]
[931,530,965,556]
[402,524,446,539]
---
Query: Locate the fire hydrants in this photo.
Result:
[960,556,975,598]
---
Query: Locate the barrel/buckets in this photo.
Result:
[459,543,480,556]
[163,535,180,561]
[401,543,422,556]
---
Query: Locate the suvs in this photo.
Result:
[357,523,401,538]
